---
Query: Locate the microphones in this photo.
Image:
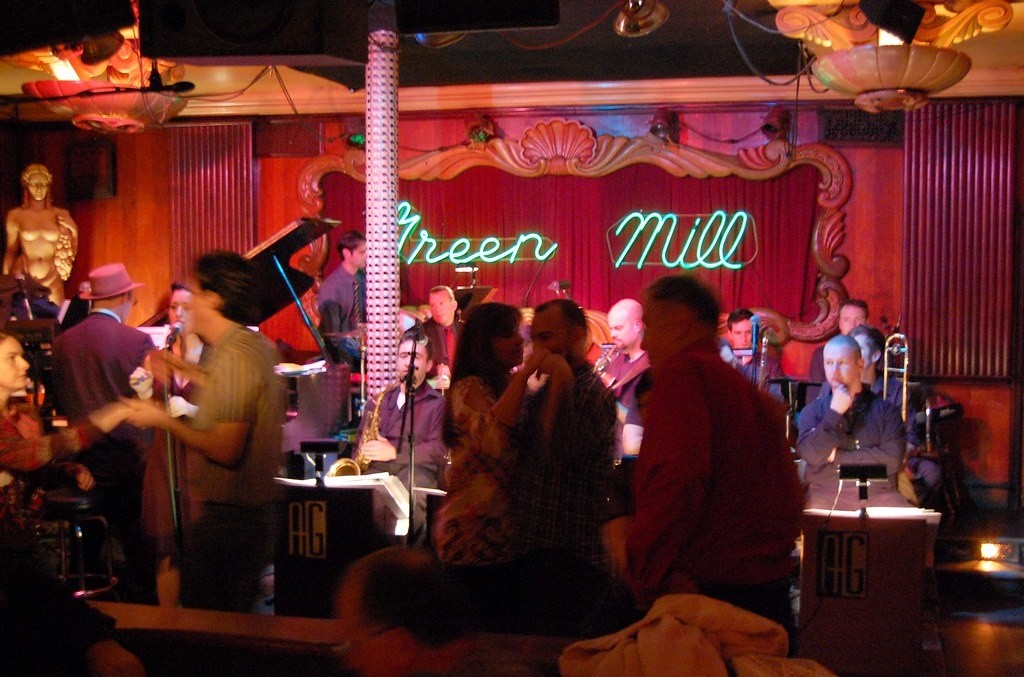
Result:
[15,273,28,299]
[166,322,184,346]
[415,317,429,347]
[749,315,761,355]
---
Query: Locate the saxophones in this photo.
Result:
[328,377,403,477]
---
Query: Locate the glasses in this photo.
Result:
[128,291,137,307]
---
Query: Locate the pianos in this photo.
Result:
[130,212,351,455]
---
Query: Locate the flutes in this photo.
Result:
[760,335,768,390]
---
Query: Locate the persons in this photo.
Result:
[1,163,79,308]
[0,231,939,677]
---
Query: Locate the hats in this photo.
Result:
[79,264,143,300]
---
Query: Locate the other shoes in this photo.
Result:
[924,568,937,601]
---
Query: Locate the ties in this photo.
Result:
[444,326,455,365]
[396,382,406,411]
[178,333,187,389]
[353,281,361,327]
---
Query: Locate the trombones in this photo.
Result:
[880,330,908,424]
